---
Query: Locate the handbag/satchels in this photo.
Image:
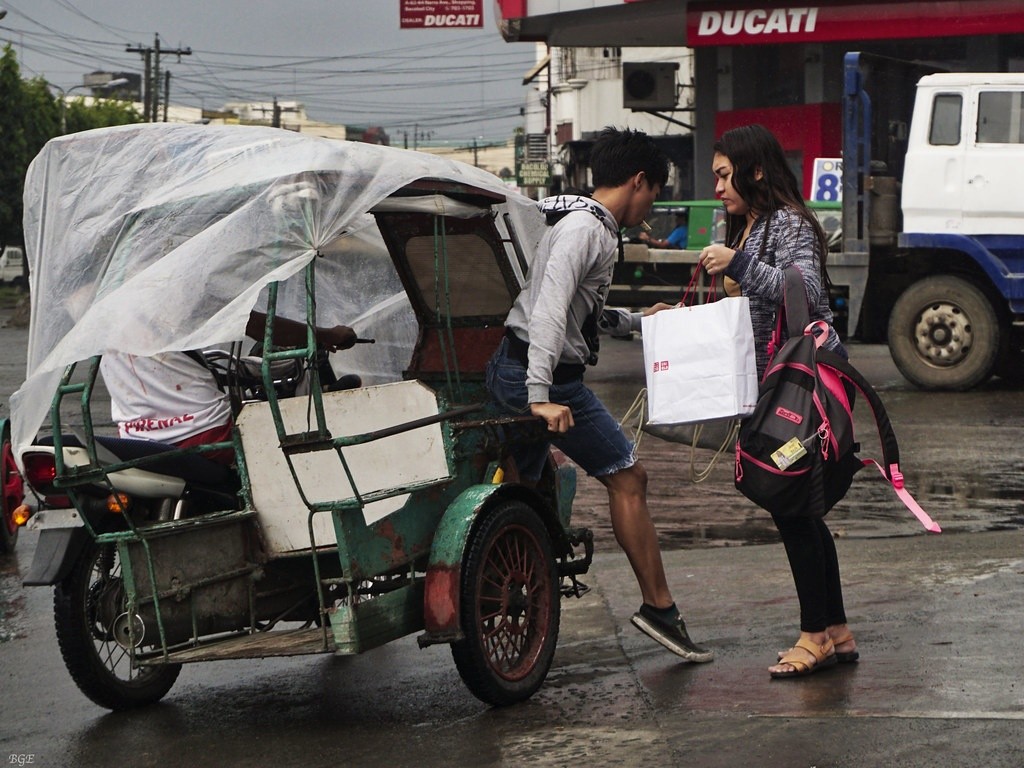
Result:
[616,380,740,483]
[640,259,759,427]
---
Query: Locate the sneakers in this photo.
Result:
[630,602,714,664]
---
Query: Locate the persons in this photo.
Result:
[97,211,358,451]
[485,127,714,663]
[639,212,689,249]
[698,125,860,680]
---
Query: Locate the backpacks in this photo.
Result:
[734,265,941,534]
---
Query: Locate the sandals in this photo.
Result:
[767,638,837,680]
[777,631,859,665]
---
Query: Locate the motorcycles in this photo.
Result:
[13,119,601,717]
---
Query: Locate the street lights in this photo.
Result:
[60,77,131,131]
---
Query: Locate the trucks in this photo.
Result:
[607,47,1024,394]
